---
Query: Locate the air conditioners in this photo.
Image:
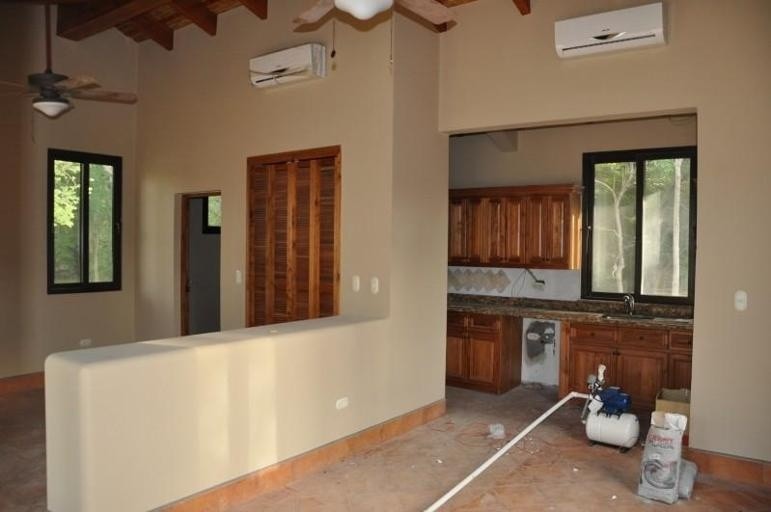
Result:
[249,42,328,90]
[555,2,669,60]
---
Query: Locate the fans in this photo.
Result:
[0,0,139,144]
[299,0,454,59]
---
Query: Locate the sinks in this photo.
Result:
[601,313,657,321]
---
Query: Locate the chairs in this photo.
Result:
[446,309,523,395]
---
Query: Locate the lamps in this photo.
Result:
[31,96,72,121]
[334,0,395,21]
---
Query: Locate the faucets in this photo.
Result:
[624,295,635,315]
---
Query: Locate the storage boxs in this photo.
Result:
[656,387,691,436]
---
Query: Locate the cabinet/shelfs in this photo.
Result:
[668,327,694,391]
[568,322,669,412]
[448,194,583,271]
